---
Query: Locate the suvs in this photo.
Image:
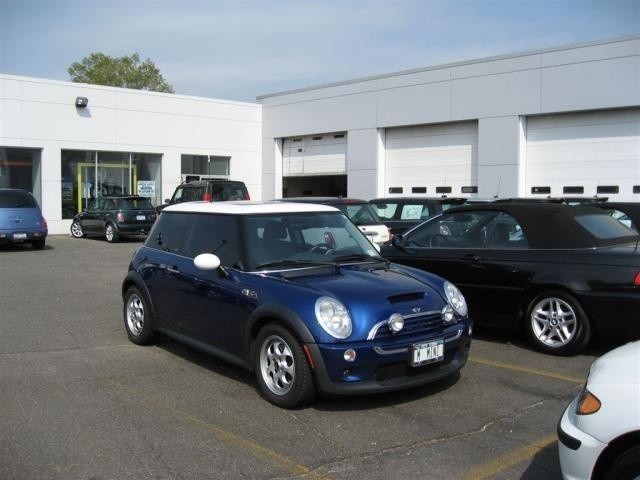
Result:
[155,180,250,218]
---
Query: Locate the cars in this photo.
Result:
[351,194,493,238]
[554,336,640,480]
[509,196,640,247]
[70,194,156,243]
[121,197,475,411]
[375,196,640,359]
[0,186,48,252]
[255,196,393,257]
[78,181,128,212]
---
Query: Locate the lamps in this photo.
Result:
[75,97,88,106]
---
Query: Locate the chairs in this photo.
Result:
[263,221,290,258]
[486,222,509,248]
